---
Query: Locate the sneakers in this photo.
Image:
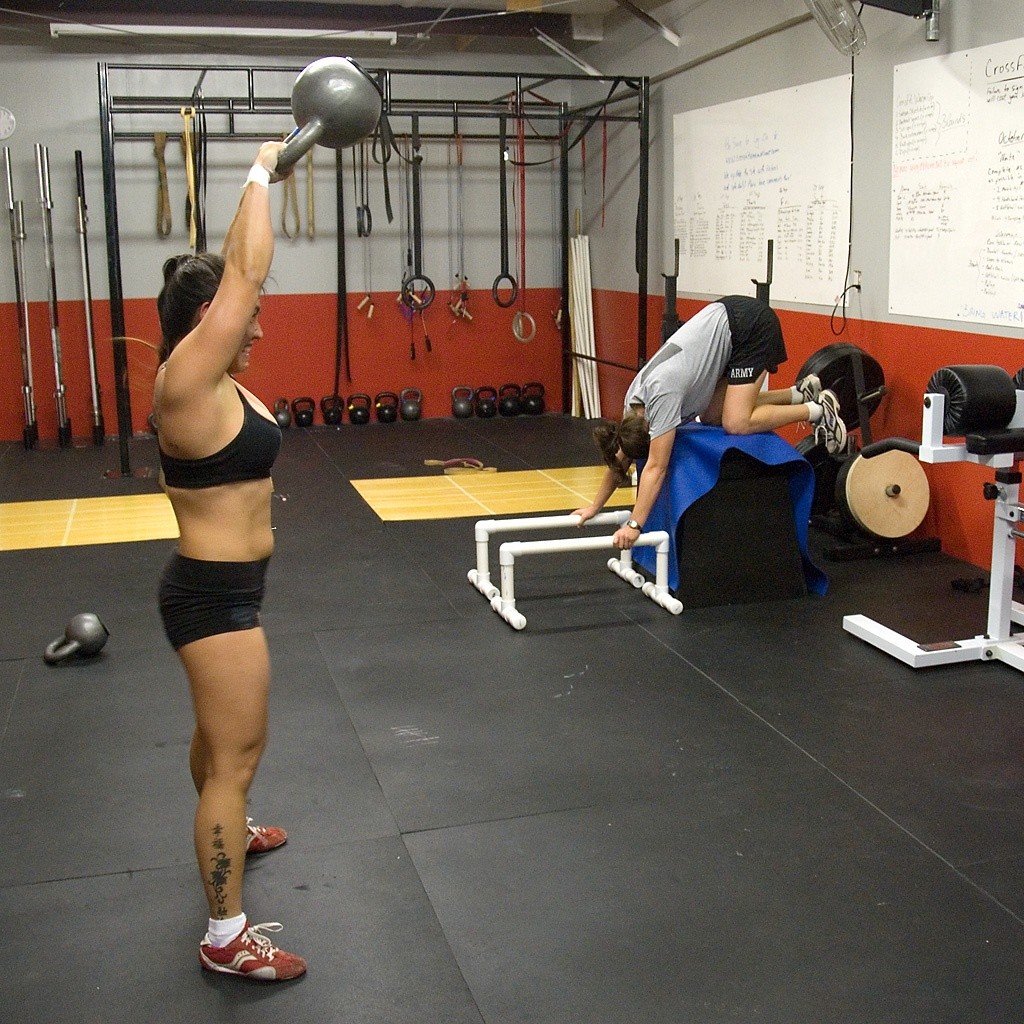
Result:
[198,917,306,981]
[794,373,821,435]
[811,389,847,455]
[243,817,288,854]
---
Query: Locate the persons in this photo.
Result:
[148,141,306,981]
[569,296,847,551]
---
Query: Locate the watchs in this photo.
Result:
[626,520,643,533]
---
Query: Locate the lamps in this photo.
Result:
[49,22,397,45]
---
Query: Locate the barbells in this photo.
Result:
[791,432,930,541]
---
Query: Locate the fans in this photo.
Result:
[803,0,942,57]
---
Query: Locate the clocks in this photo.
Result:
[0,106,16,140]
